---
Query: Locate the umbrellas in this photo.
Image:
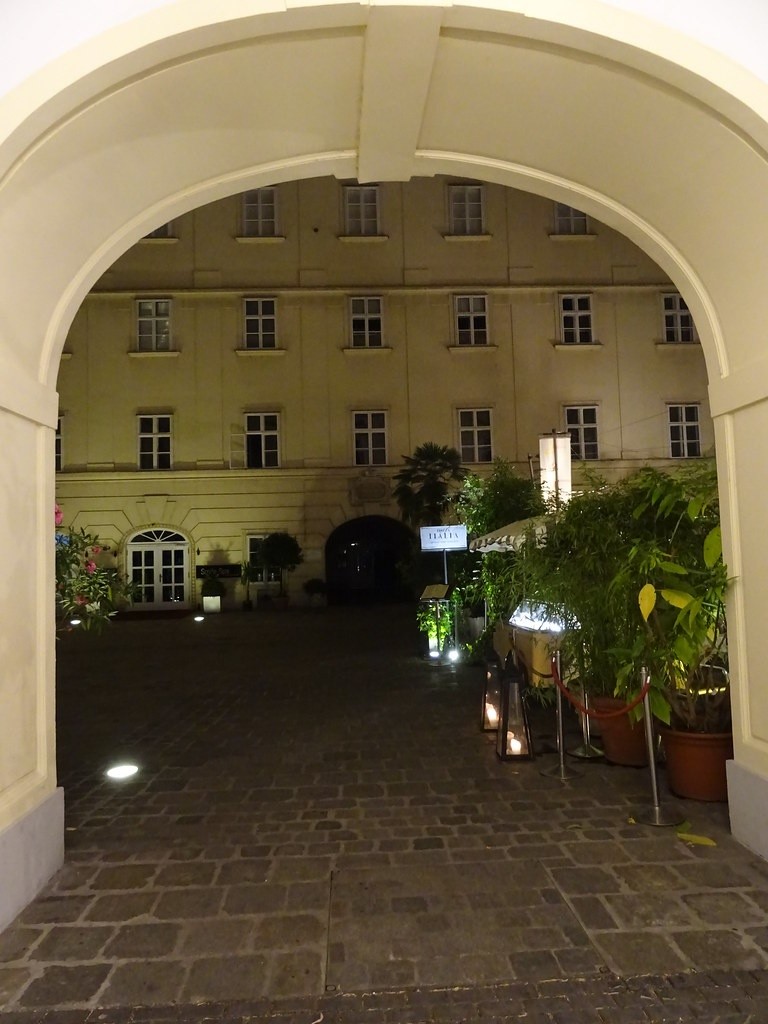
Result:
[469,514,566,617]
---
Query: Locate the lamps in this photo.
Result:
[495,648,534,760]
[481,647,503,733]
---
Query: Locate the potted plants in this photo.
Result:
[238,560,257,612]
[301,577,329,606]
[482,456,734,800]
[254,533,304,611]
[202,575,226,614]
